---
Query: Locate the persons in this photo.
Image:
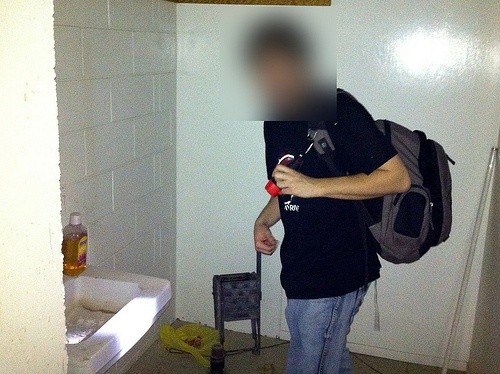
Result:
[244,22,413,374]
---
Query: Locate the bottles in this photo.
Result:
[63,212,86,276]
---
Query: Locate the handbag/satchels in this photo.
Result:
[158,320,219,368]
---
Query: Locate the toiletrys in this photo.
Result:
[62,211,88,276]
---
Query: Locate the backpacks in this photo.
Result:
[307,90,456,267]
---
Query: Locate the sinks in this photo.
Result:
[63,264,173,374]
